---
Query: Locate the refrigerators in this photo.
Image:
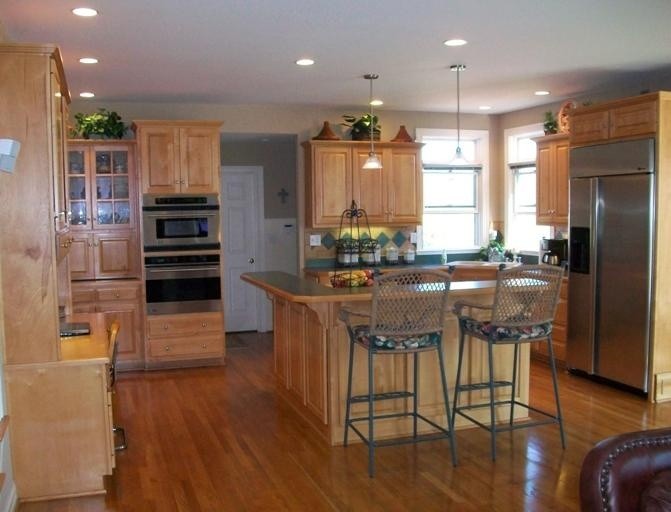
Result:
[567,138,659,395]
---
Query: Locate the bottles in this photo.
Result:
[442,248,448,264]
[386,237,399,265]
[404,238,416,264]
[336,240,382,266]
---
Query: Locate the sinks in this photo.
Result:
[451,259,519,267]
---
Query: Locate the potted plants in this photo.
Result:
[340,113,381,141]
[478,241,505,262]
[544,111,560,136]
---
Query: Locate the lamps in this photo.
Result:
[450,63,471,172]
[362,74,382,169]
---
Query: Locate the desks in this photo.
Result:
[239,272,552,446]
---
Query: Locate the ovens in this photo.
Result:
[141,210,220,250]
[143,264,222,312]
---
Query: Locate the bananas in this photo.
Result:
[342,271,368,287]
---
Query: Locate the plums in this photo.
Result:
[365,270,374,286]
[331,276,345,288]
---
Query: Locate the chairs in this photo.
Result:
[452,259,570,464]
[340,269,456,479]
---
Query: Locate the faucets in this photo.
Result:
[488,246,498,263]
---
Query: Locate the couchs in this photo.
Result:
[579,428,671,512]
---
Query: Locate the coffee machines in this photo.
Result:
[542,239,563,266]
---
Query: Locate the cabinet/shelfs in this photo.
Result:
[517,272,569,370]
[306,267,499,287]
[530,133,572,225]
[136,118,226,194]
[570,91,671,147]
[144,298,227,370]
[301,140,425,228]
